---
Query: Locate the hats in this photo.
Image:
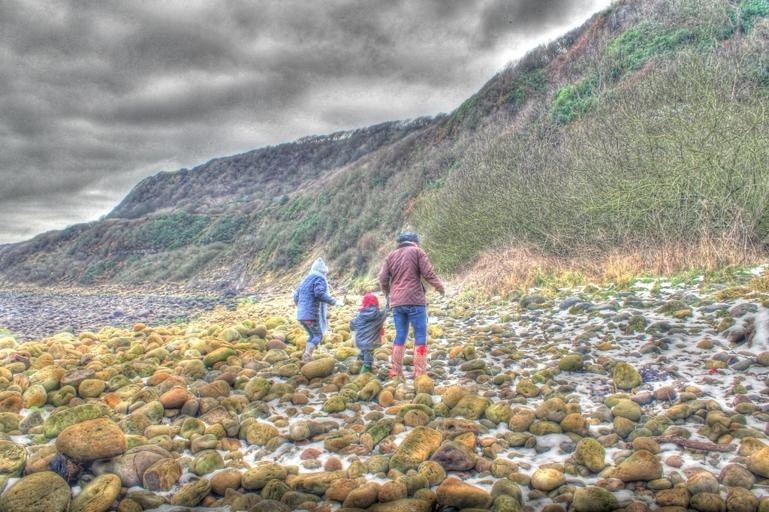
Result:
[363,293,379,307]
[395,231,419,243]
[310,257,329,274]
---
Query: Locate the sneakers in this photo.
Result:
[360,364,371,374]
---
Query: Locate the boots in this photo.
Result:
[387,344,407,379]
[411,345,428,379]
[302,342,316,361]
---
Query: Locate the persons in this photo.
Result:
[294,256,342,363]
[379,230,444,381]
[352,294,389,375]
